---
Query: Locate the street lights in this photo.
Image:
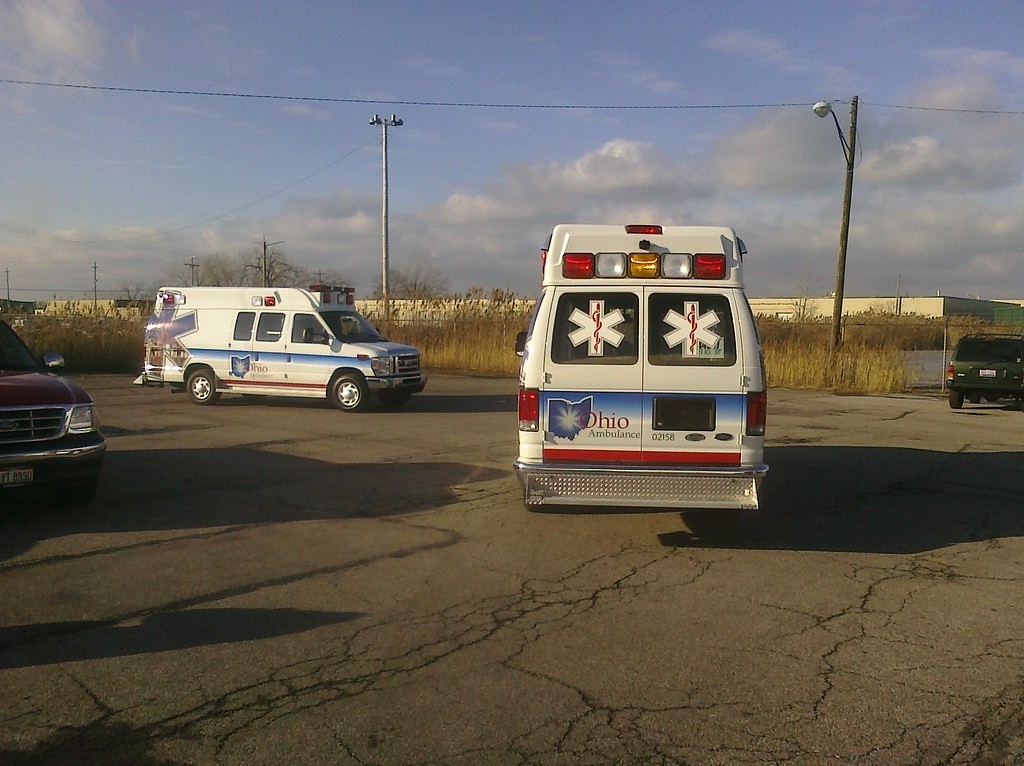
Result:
[367,114,404,341]
[812,96,859,360]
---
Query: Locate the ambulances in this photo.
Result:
[516,223,768,511]
[132,286,427,412]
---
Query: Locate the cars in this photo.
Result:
[0,318,106,509]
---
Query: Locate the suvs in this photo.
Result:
[944,334,1024,410]
